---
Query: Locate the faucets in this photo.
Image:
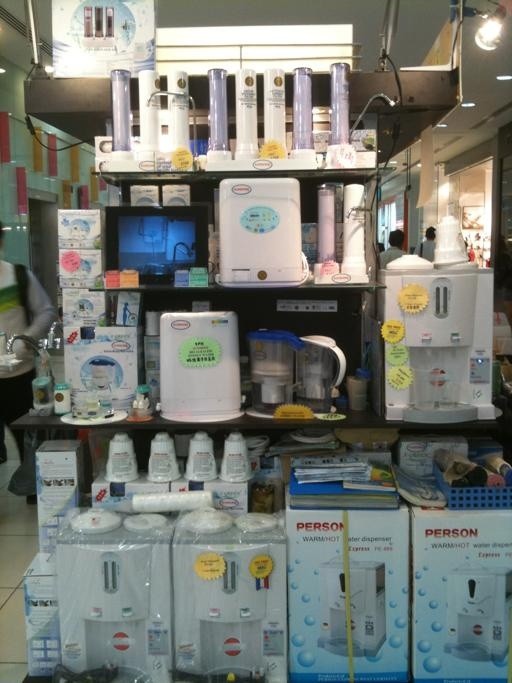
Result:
[46,321,58,351]
[4,334,40,354]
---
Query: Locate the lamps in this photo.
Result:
[463,1,507,52]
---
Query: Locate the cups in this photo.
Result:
[32,377,52,410]
[247,329,303,415]
[294,336,346,415]
[70,380,114,418]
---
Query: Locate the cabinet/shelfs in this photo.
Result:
[9,69,512,427]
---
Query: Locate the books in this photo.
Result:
[389,461,447,508]
[290,451,373,486]
[340,461,397,494]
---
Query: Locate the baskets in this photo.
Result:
[432,456,512,509]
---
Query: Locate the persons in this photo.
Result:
[0,222,60,507]
[413,225,436,262]
[376,228,409,268]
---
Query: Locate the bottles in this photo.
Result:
[135,384,153,413]
[53,384,71,415]
[434,212,470,267]
